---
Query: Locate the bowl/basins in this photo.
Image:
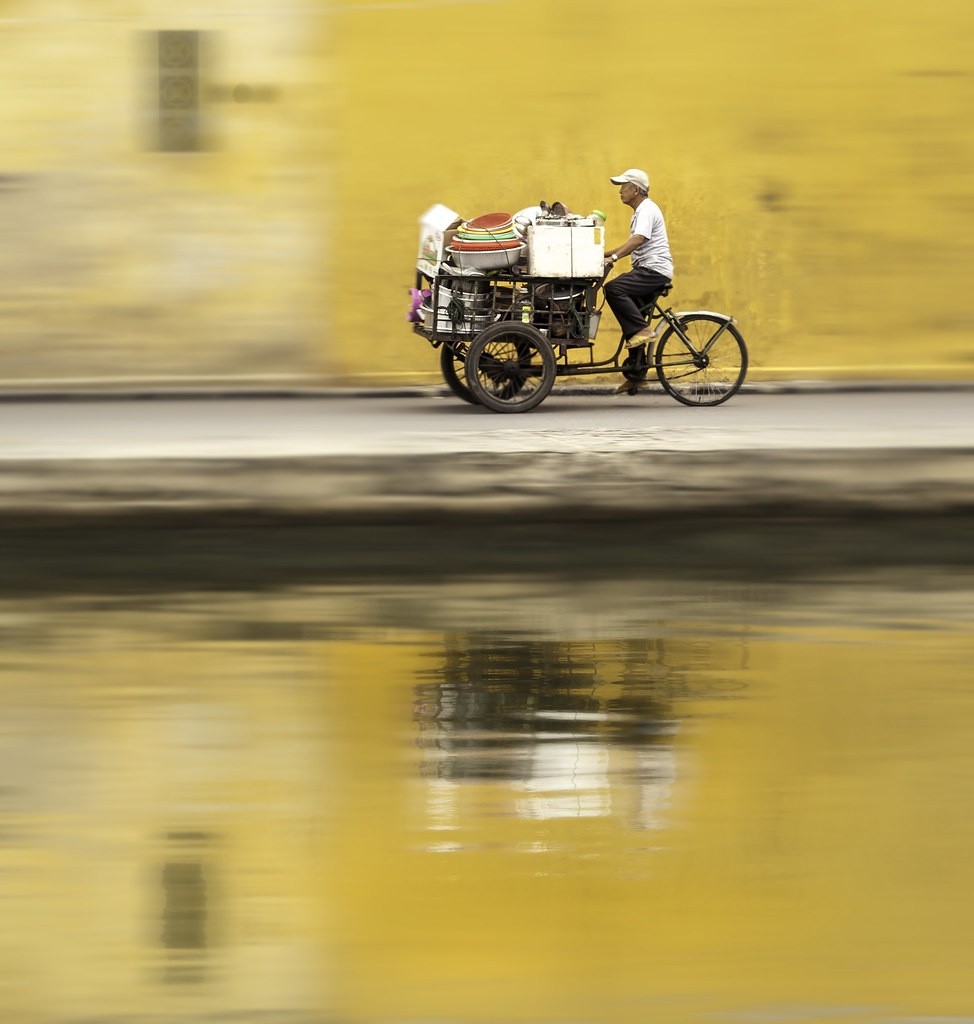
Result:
[445,212,528,270]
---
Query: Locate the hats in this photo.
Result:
[611,169,649,194]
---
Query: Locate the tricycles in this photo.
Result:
[410,257,748,413]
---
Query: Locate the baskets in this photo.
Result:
[451,212,520,251]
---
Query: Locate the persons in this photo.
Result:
[604,168,674,395]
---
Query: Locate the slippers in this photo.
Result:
[613,380,649,394]
[624,334,656,348]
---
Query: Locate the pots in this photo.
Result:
[534,283,582,311]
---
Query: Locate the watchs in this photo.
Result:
[612,254,617,262]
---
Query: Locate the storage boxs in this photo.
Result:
[527,226,605,278]
[418,204,464,278]
[588,308,602,339]
